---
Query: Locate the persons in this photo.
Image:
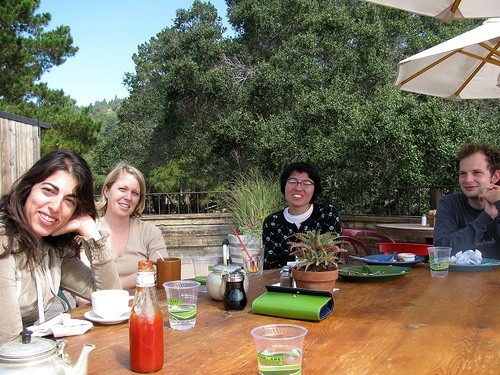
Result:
[261,162,343,271]
[433,145,500,261]
[0,150,123,346]
[75,163,169,309]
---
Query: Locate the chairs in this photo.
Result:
[342,228,396,263]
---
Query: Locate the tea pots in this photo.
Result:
[0,331,97,375]
[206,238,249,301]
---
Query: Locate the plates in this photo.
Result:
[339,265,413,283]
[362,255,424,266]
[83,307,132,325]
[428,257,500,271]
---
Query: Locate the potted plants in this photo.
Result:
[285,230,352,293]
[213,148,288,266]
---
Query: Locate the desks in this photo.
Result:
[376,224,434,243]
[62,259,500,375]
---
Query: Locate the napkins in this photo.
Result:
[26,313,94,337]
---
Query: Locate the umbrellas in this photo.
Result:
[367,0,500,21]
[395,18,500,102]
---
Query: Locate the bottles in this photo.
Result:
[222,271,247,311]
[280,266,293,287]
[422,214,427,226]
[129,260,164,374]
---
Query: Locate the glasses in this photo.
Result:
[287,180,315,188]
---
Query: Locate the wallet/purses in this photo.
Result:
[252,284,334,322]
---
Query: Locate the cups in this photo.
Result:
[163,280,202,331]
[250,323,308,375]
[427,246,452,278]
[397,254,416,261]
[91,289,134,319]
[156,256,181,288]
